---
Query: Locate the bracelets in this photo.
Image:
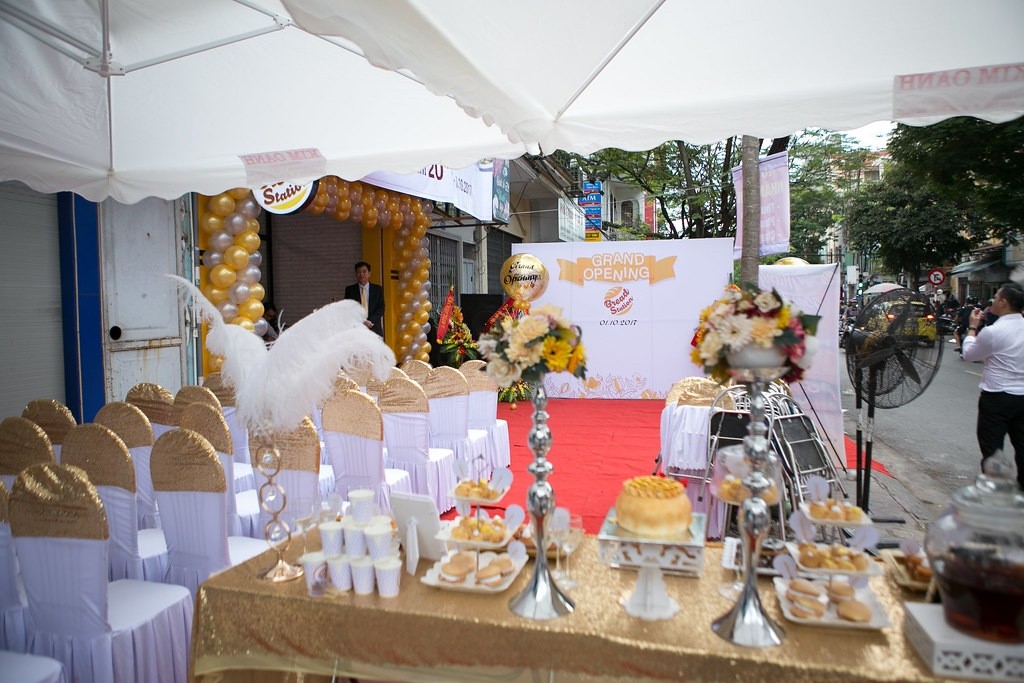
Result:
[966,327,976,331]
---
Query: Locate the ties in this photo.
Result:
[362,287,368,312]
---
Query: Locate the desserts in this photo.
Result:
[810,499,862,520]
[450,514,506,542]
[438,553,514,589]
[907,548,936,581]
[454,478,497,499]
[786,577,872,621]
[798,541,867,570]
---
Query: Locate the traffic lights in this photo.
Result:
[857,273,863,295]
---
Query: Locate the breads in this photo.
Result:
[666,377,735,409]
[503,525,559,550]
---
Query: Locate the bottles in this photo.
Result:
[923,449,1024,646]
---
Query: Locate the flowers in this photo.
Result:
[691,281,810,386]
[478,310,586,399]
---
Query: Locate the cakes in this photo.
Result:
[719,472,778,504]
[615,476,690,536]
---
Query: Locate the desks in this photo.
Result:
[188,517,984,683]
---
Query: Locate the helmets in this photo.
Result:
[966,295,974,303]
[973,297,978,304]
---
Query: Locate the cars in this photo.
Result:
[874,300,937,348]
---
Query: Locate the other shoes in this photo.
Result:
[954,347,961,351]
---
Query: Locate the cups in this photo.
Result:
[298,488,403,598]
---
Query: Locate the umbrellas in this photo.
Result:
[862,283,905,295]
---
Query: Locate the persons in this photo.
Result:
[842,298,862,330]
[962,284,1024,493]
[927,294,998,353]
[344,262,385,338]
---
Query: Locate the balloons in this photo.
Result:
[200,175,433,376]
[499,253,549,309]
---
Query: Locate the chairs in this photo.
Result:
[0,356,510,683]
[651,374,850,542]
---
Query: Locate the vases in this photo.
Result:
[725,344,787,367]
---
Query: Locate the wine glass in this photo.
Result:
[290,497,314,565]
[525,508,583,588]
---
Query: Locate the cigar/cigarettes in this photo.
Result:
[973,307,984,314]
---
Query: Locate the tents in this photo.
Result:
[0,0,1024,205]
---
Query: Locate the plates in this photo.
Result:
[721,536,828,580]
[877,547,937,593]
[479,525,586,559]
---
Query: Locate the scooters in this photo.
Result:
[938,308,970,360]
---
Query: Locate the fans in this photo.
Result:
[843,288,944,548]
